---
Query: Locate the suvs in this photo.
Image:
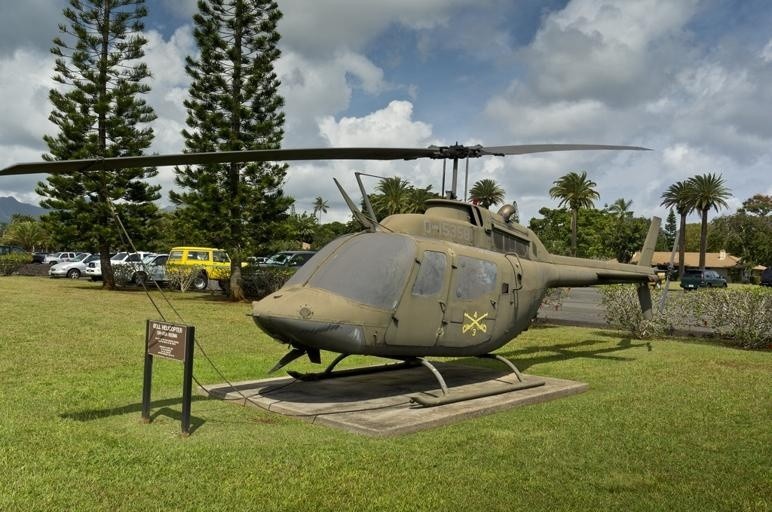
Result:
[164,246,248,290]
[241,250,317,298]
[680,268,727,292]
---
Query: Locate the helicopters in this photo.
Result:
[0,143,681,407]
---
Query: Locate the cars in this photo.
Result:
[0,244,157,281]
[124,254,209,289]
[246,256,269,264]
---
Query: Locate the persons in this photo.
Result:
[758,256,771,287]
